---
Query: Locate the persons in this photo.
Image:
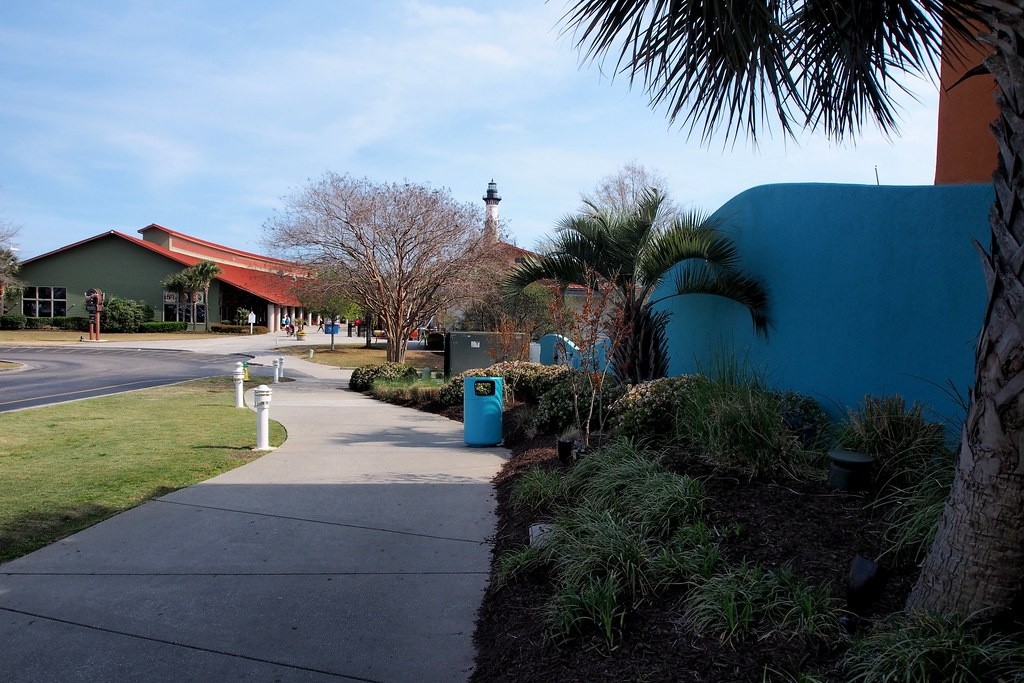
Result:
[282,316,324,337]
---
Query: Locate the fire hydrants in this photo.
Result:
[243,360,251,382]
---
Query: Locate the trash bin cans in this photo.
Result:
[464,376,503,446]
